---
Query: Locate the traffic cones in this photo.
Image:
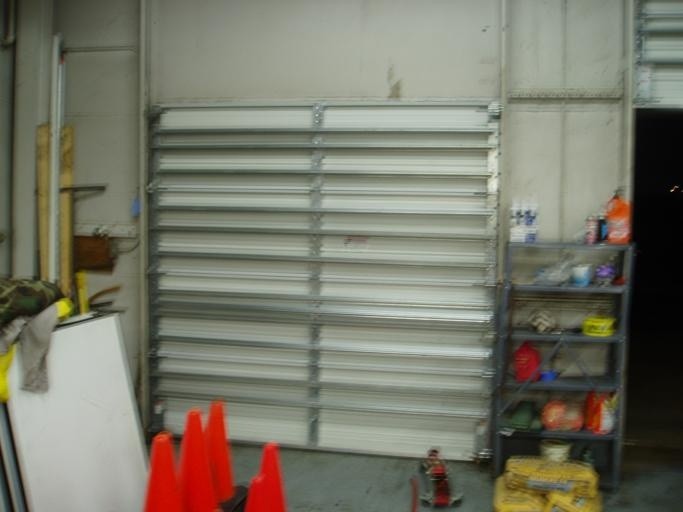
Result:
[141,400,287,511]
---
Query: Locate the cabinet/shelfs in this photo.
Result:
[490,241,633,495]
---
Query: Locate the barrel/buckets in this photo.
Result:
[539,439,571,460]
[605,190,631,244]
[514,344,540,382]
